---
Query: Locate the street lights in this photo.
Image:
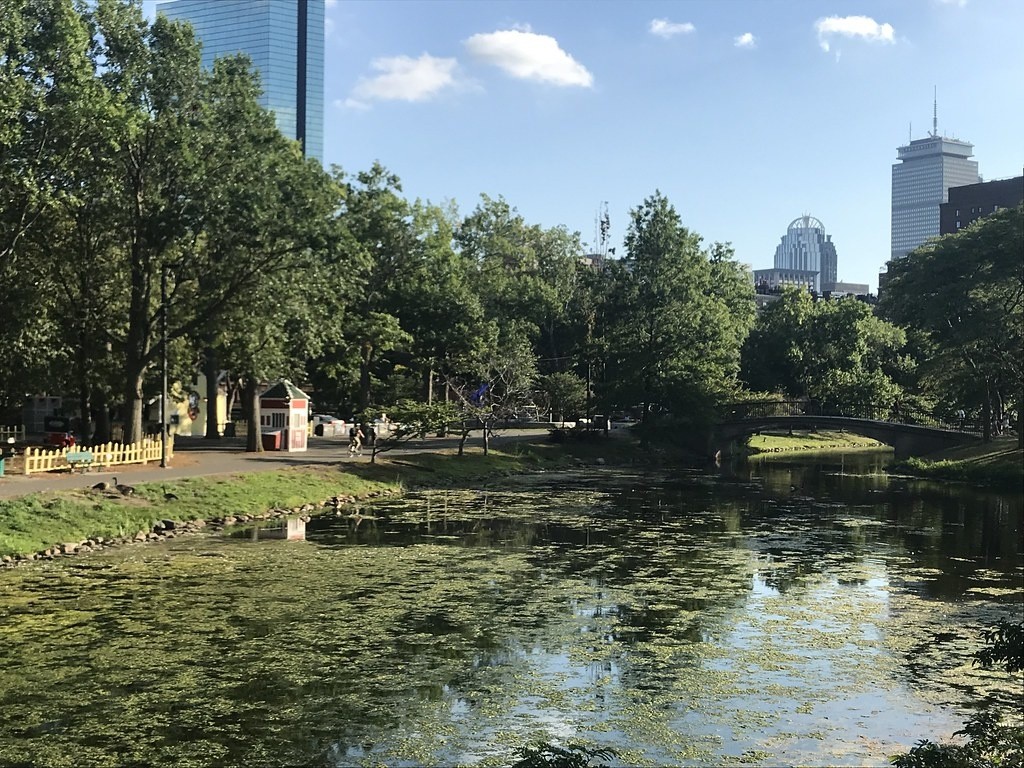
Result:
[159,260,185,470]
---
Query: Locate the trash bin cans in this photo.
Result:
[226,423,236,437]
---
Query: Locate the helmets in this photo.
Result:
[356,423,361,426]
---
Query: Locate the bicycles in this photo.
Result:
[346,435,367,460]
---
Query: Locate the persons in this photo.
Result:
[349,423,365,453]
[61,431,75,450]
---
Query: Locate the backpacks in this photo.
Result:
[350,428,361,437]
